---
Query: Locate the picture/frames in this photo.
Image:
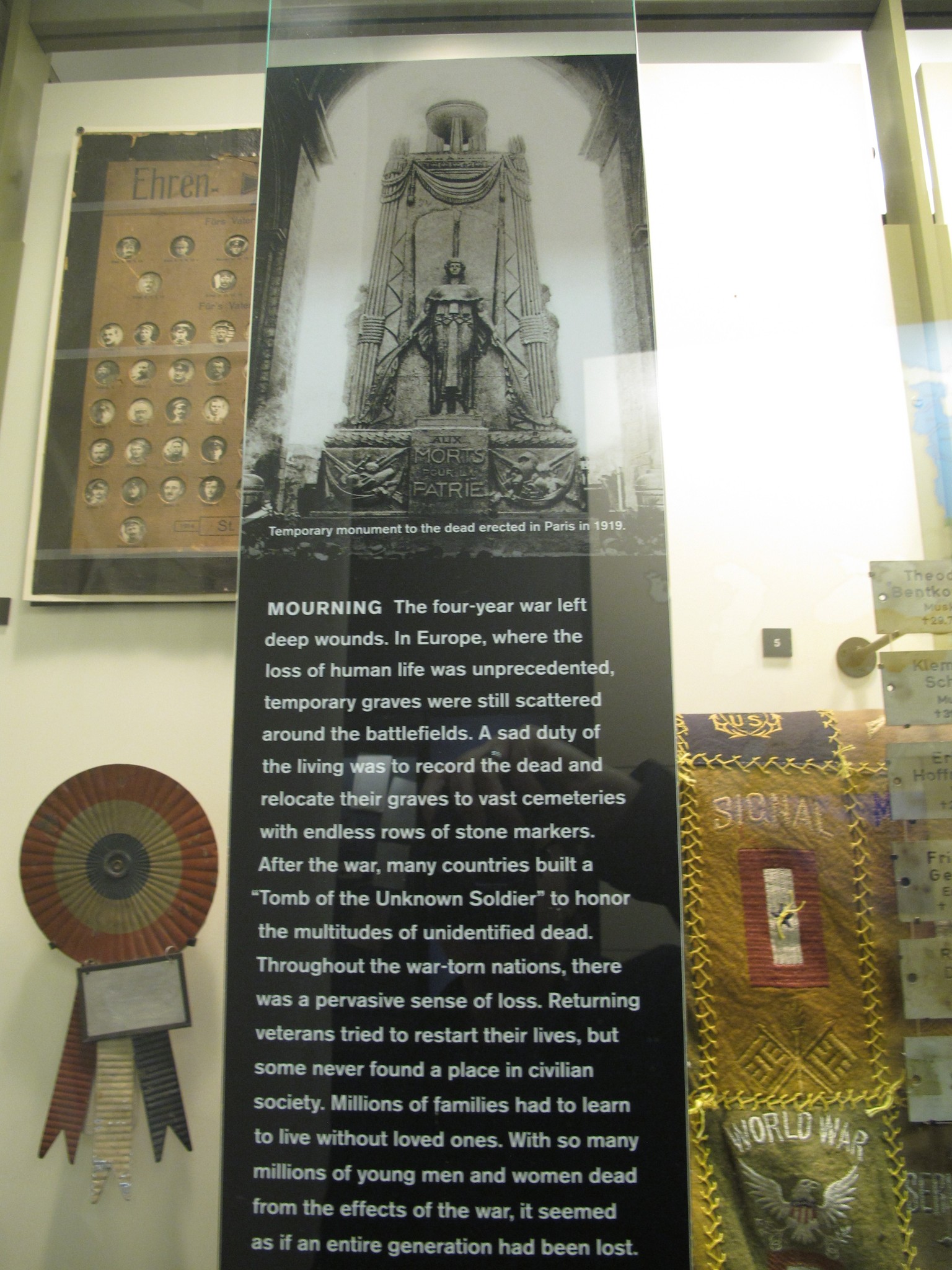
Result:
[77,951,192,1044]
[18,126,265,605]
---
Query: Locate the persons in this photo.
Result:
[216,270,234,293]
[406,258,496,413]
[142,273,157,294]
[86,318,235,544]
[229,238,242,254]
[121,238,137,259]
[174,237,189,258]
